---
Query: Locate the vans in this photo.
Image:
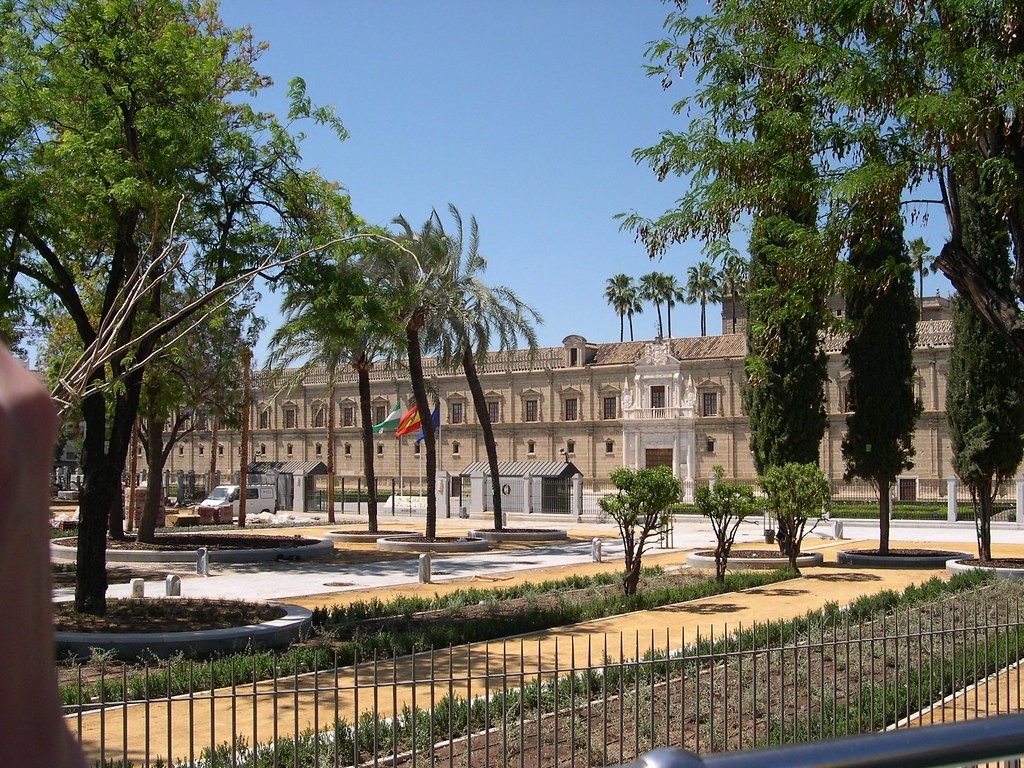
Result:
[199,485,278,516]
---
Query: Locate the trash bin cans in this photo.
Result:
[466,507,473,518]
[764,530,775,544]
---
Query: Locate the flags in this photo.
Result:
[372,401,401,433]
[416,400,440,444]
[394,404,421,440]
